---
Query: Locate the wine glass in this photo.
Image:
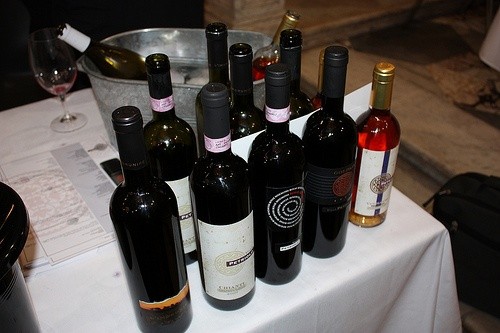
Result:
[26,27,88,132]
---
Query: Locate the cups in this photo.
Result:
[0,179,44,333]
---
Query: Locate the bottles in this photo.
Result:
[54,22,149,81]
[187,81,258,311]
[108,105,193,333]
[194,9,324,143]
[301,45,358,259]
[143,53,199,265]
[348,62,401,228]
[246,62,305,286]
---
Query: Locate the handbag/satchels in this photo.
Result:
[421,172,500,321]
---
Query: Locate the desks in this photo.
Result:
[0,87,462,333]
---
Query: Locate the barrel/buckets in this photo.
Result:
[76,29,272,155]
